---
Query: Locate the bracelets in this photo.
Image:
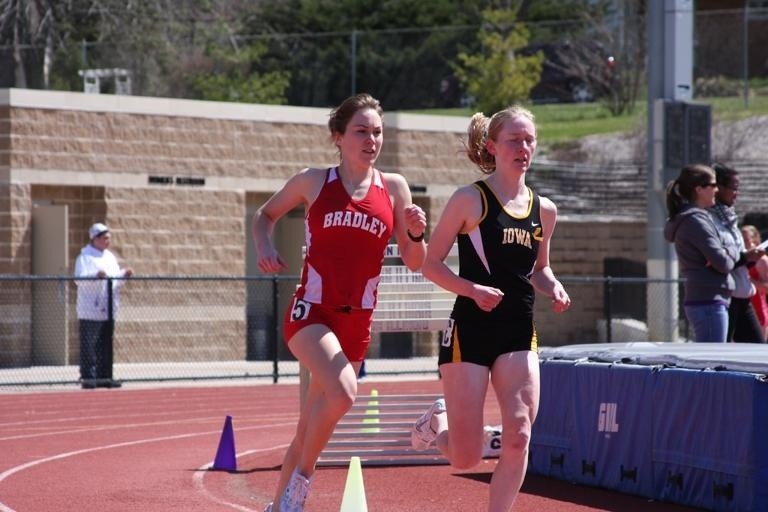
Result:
[407,230,424,243]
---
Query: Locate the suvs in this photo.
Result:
[436,34,614,115]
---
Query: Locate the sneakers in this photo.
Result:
[263,501,273,512]
[97,378,122,388]
[80,378,97,389]
[410,397,446,452]
[278,464,317,512]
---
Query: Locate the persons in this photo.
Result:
[251,94,428,512]
[665,165,768,342]
[411,106,572,512]
[74,222,134,389]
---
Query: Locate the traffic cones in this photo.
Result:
[332,453,371,512]
[356,387,382,433]
[210,413,241,473]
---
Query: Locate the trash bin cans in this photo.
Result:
[73,67,133,95]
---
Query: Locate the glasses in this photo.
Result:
[704,181,718,187]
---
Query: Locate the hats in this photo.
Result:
[88,222,109,241]
